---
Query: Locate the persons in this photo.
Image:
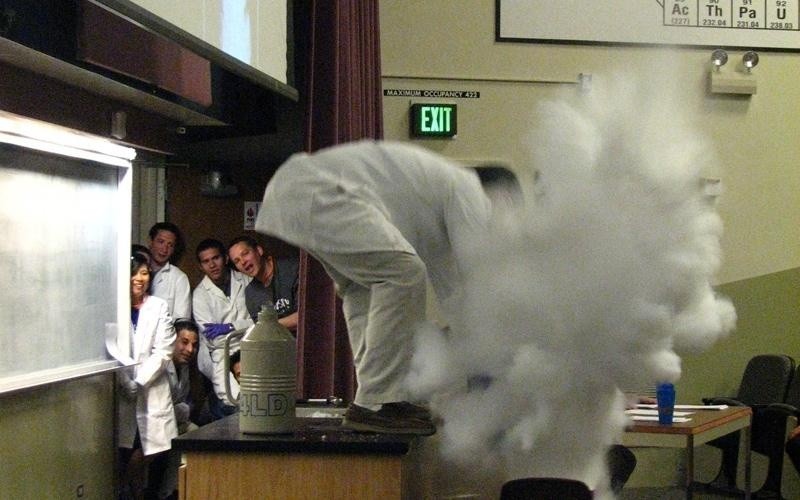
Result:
[147,222,192,327]
[167,321,200,500]
[192,237,256,419]
[228,235,298,329]
[254,139,515,435]
[116,253,178,500]
[131,244,152,294]
[228,350,241,387]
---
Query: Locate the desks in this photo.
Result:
[172,396,421,500]
[591,399,754,498]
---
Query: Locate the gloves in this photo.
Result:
[201,322,234,339]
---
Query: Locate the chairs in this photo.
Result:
[696,354,797,499]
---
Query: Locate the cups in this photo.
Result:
[656,382,676,425]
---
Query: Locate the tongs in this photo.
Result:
[296,395,344,408]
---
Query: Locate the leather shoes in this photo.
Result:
[341,401,436,437]
[378,400,431,418]
